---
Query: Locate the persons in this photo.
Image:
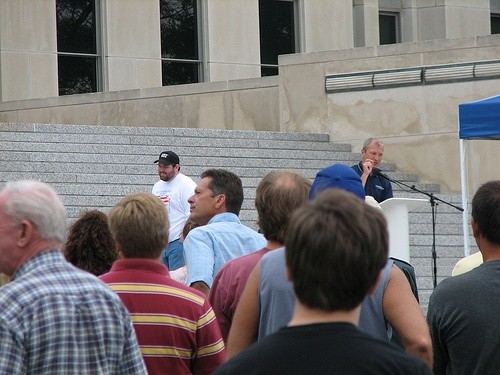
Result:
[0,179,149,375]
[427,181,500,375]
[212,187,433,375]
[63,210,119,277]
[182,217,200,241]
[350,138,394,203]
[97,193,227,375]
[152,151,199,272]
[226,163,435,372]
[182,169,269,296]
[206,169,312,346]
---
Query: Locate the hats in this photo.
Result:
[153,150,180,166]
[310,163,366,202]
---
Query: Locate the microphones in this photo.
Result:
[372,166,379,175]
